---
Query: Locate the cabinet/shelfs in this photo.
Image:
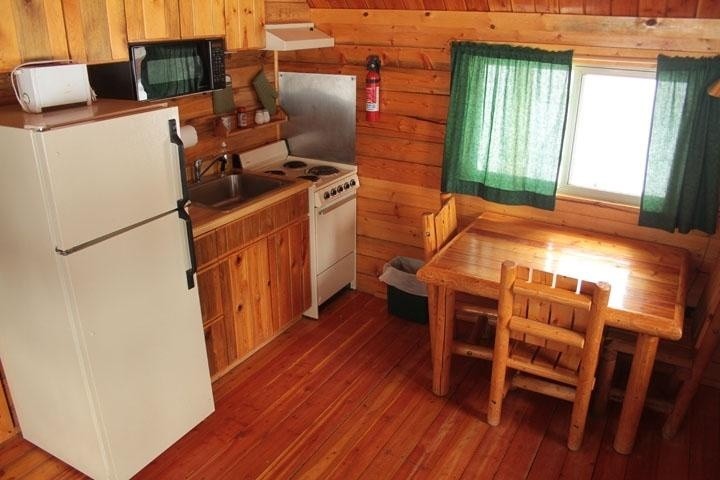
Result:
[193,189,312,381]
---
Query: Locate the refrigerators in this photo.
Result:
[1,98,217,480]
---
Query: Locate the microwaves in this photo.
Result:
[128,34,227,103]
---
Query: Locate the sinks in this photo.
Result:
[189,168,295,210]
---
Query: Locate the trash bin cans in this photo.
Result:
[383,255,429,324]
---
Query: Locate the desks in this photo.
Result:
[416,211,691,455]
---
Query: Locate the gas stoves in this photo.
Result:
[260,156,357,194]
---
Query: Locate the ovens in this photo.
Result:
[315,172,360,304]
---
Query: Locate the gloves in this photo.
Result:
[213,74,237,114]
[253,71,278,110]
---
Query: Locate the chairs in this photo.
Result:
[487,262,611,451]
[422,193,492,368]
[596,259,720,441]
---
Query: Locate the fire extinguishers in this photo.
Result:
[365,55,380,122]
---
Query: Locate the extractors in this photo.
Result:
[258,22,335,51]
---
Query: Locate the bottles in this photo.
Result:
[236,106,249,128]
[263,108,271,124]
[254,109,264,124]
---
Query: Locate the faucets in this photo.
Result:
[191,153,228,182]
